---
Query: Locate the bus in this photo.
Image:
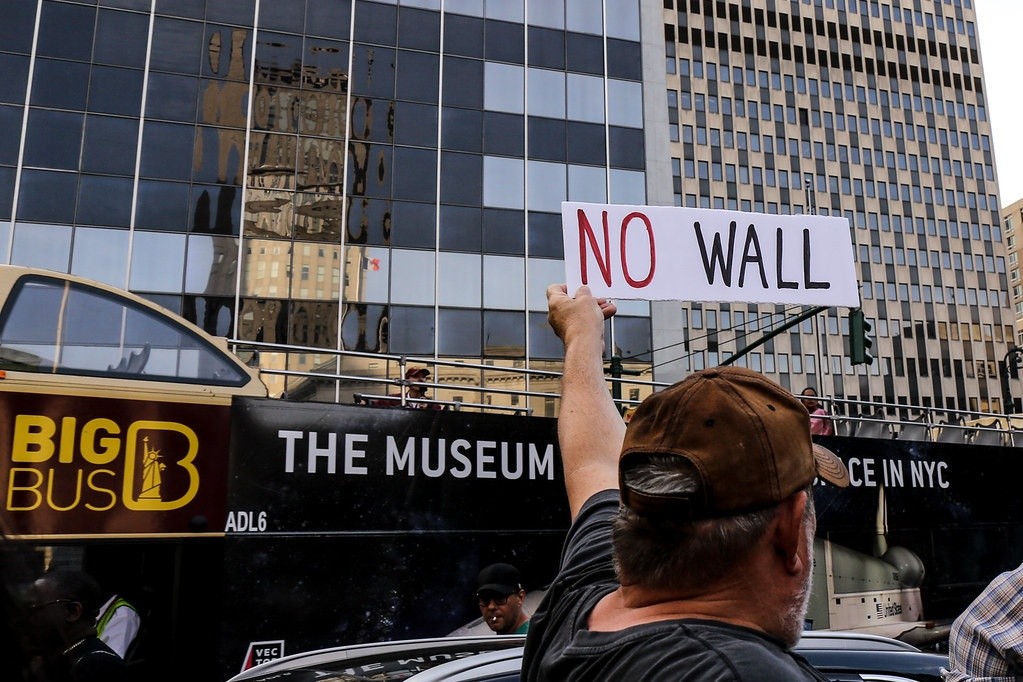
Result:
[0,264,1023,682]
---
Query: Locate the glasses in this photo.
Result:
[476,589,520,607]
[25,597,72,621]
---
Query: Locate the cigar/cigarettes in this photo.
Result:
[492,617,497,621]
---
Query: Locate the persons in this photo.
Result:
[359,366,441,410]
[938,561,1023,682]
[0,568,160,682]
[800,387,833,435]
[521,283,853,682]
[475,565,530,635]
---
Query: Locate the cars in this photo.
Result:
[228,631,947,682]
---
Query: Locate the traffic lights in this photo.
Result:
[849,310,874,367]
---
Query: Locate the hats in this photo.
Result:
[477,563,522,592]
[618,366,850,520]
